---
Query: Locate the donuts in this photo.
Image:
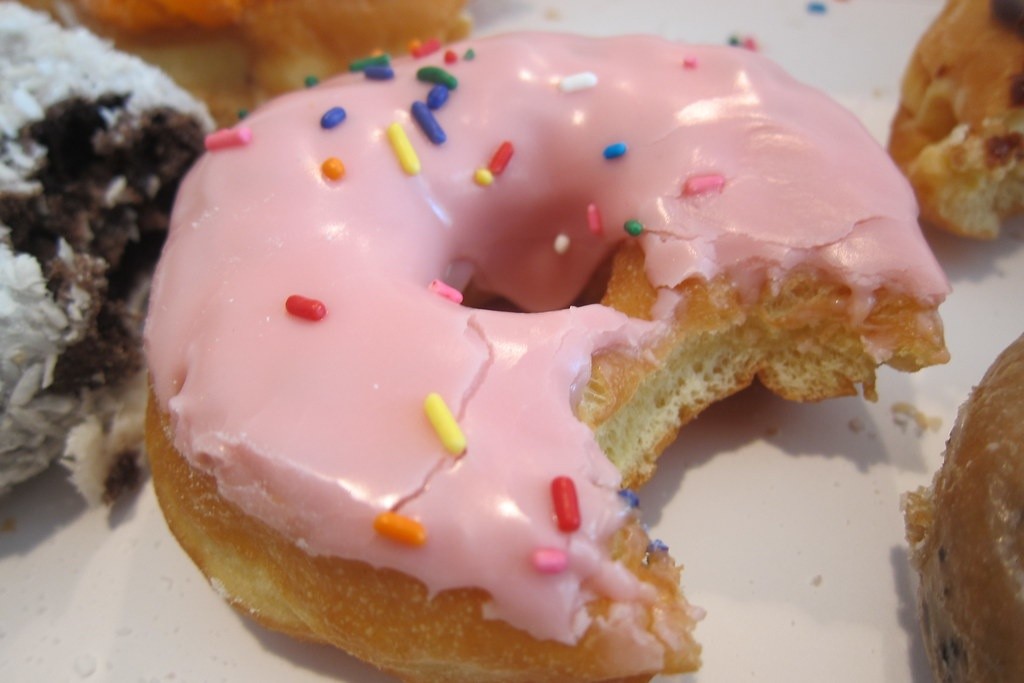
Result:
[0,0,1024,683]
